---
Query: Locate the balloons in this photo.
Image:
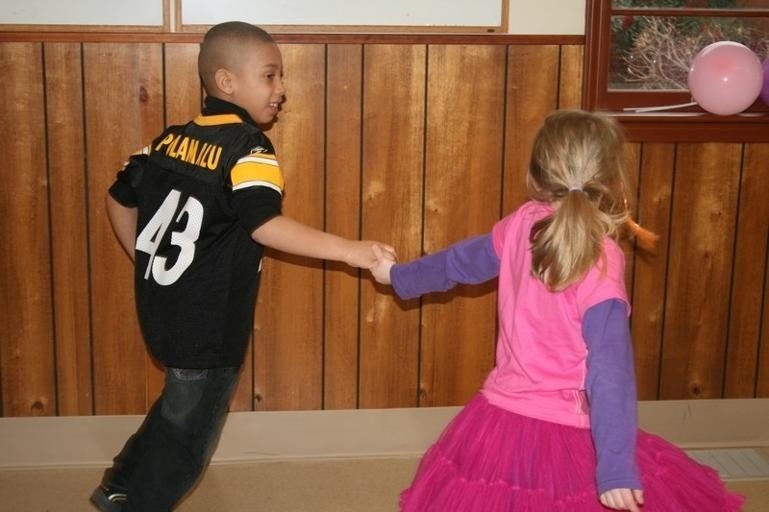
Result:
[689,39,763,116]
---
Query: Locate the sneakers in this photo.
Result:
[88,464,131,510]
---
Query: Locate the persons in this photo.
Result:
[368,109,748,512]
[89,20,399,512]
